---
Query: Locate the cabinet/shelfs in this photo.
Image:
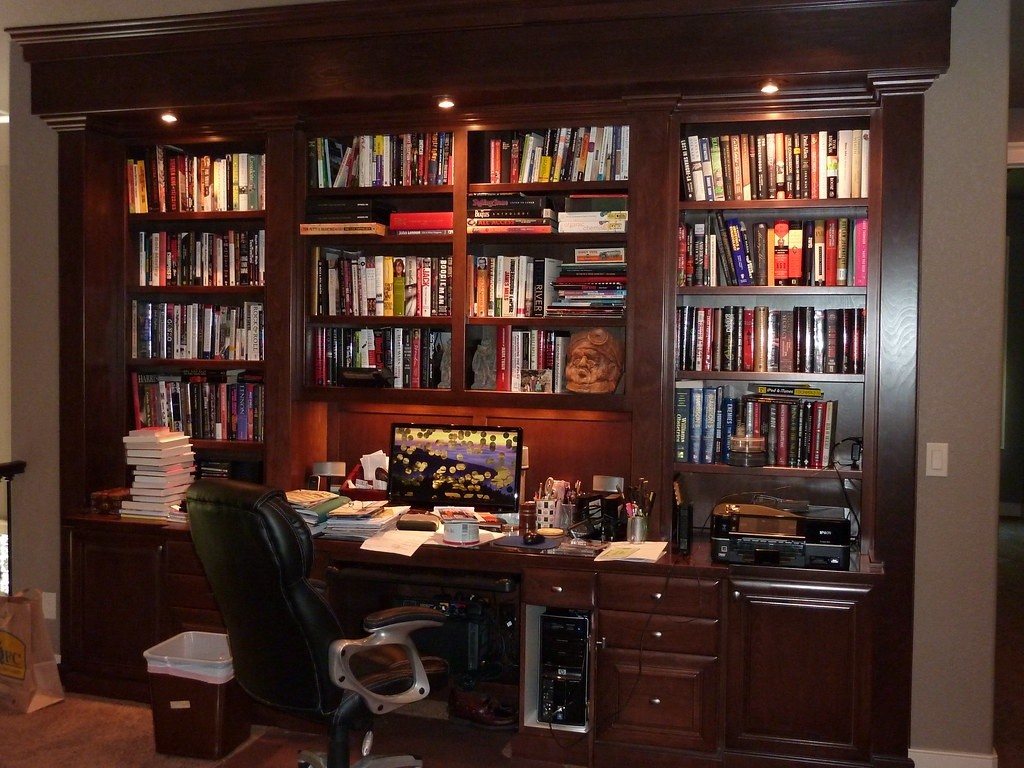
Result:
[12,0,953,766]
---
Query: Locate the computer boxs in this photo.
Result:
[536,607,591,728]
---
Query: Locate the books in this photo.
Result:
[677,500,693,554]
[118,145,268,525]
[283,488,411,542]
[298,124,631,395]
[594,541,668,563]
[670,127,872,474]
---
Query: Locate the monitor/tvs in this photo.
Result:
[386,422,523,513]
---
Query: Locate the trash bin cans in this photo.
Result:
[143,631,253,762]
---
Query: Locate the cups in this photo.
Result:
[626,516,647,542]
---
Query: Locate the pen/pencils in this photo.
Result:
[532,482,546,500]
[562,480,583,505]
[617,477,655,518]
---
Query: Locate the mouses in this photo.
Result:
[524,532,544,546]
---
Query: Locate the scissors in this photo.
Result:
[544,477,558,501]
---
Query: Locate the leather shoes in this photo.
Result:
[442,693,518,730]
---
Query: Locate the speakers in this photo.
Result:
[312,462,346,477]
[592,475,624,493]
